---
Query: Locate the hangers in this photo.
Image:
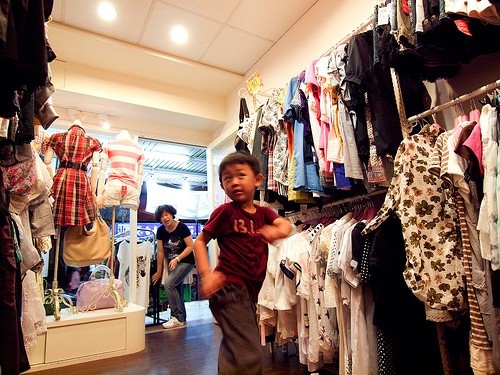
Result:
[127,14,499,241]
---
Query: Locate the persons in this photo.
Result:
[193,151,293,375]
[42,118,104,228]
[152,204,196,328]
[98,130,144,211]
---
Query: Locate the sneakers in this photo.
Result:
[162,315,185,329]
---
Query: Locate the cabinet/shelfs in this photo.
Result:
[20,304,146,375]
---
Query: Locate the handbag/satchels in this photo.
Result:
[62,201,111,266]
[76,265,125,312]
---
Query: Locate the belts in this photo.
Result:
[60,160,88,172]
